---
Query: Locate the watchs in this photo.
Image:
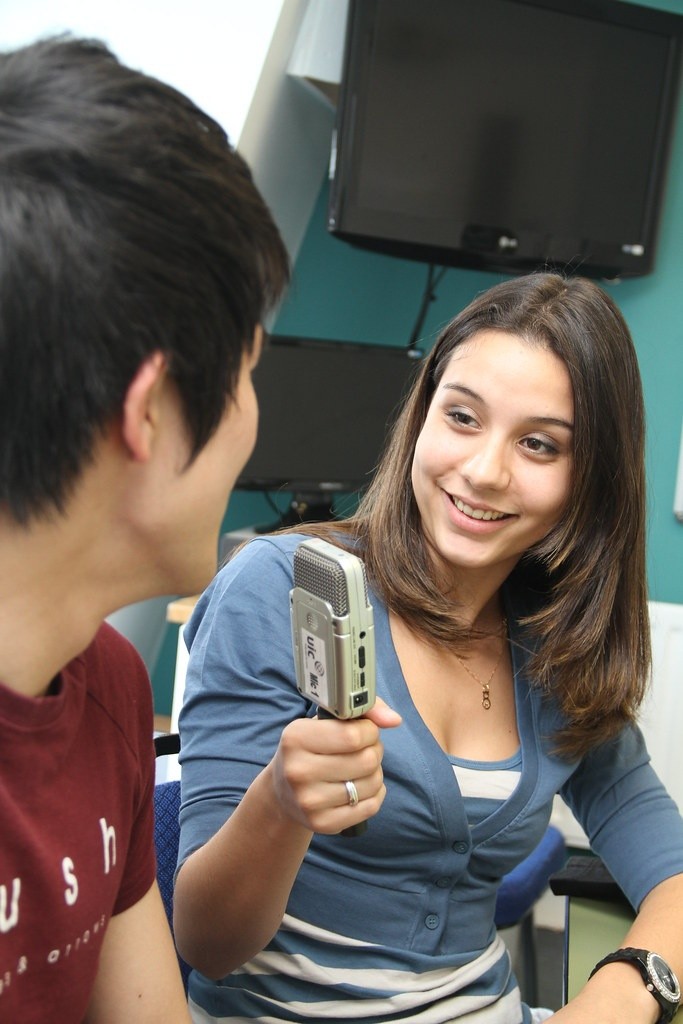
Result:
[587,945,683,1024]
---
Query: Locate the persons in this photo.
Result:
[0,27,297,1024]
[172,251,683,1024]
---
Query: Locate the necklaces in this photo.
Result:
[435,615,510,710]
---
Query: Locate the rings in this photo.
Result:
[344,780,360,805]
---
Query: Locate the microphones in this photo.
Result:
[289,537,378,834]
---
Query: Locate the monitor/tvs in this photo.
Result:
[328,0,683,280]
[237,333,424,535]
